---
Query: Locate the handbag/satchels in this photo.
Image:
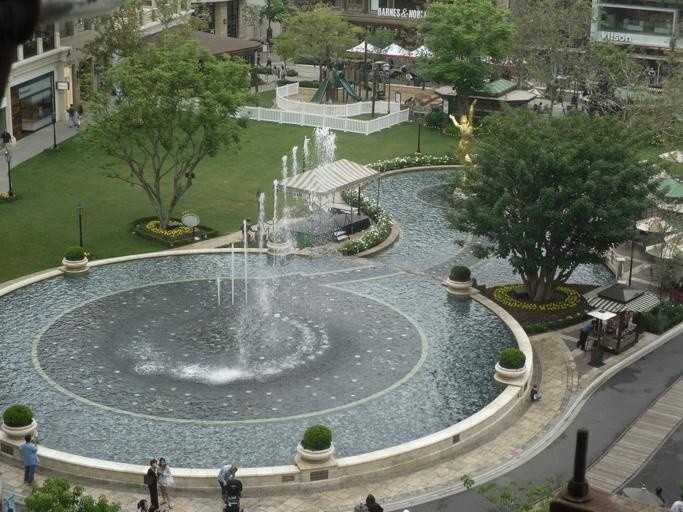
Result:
[144,475,154,484]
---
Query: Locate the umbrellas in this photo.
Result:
[344,40,435,61]
[631,146,682,265]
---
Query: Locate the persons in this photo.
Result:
[670,493,682,512]
[240,216,255,244]
[0,127,12,154]
[76,105,84,128]
[651,486,665,508]
[255,50,286,81]
[217,464,237,500]
[136,499,149,512]
[20,434,38,485]
[447,98,479,168]
[530,383,541,401]
[158,457,174,509]
[575,319,595,351]
[146,459,161,512]
[65,104,76,128]
[532,102,543,114]
[364,493,384,511]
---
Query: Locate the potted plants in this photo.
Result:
[296,425,335,464]
[1,404,38,440]
[266,229,291,253]
[62,245,88,270]
[445,265,473,292]
[494,348,529,380]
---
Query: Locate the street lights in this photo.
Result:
[256,187,261,217]
[5,150,12,194]
[50,111,56,149]
[415,114,424,152]
[628,222,639,286]
[76,202,83,248]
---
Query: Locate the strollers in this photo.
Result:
[223,479,242,512]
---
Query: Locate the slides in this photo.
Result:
[310,78,329,103]
[338,76,362,102]
